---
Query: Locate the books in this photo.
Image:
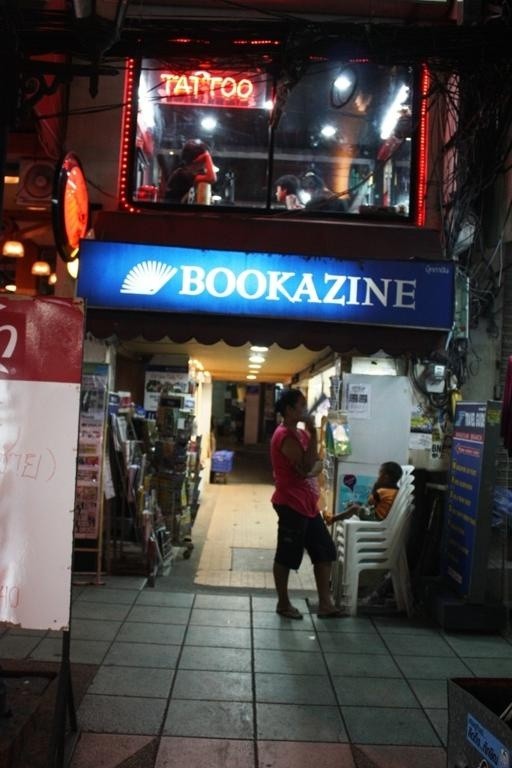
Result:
[75,374,202,564]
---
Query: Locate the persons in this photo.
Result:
[270,390,347,619]
[273,173,304,212]
[322,461,402,526]
[161,139,218,202]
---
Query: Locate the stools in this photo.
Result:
[331,464,419,620]
[213,472,226,484]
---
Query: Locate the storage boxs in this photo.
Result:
[211,449,234,473]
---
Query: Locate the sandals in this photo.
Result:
[317,610,348,617]
[277,607,301,619]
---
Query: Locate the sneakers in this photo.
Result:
[323,506,332,525]
